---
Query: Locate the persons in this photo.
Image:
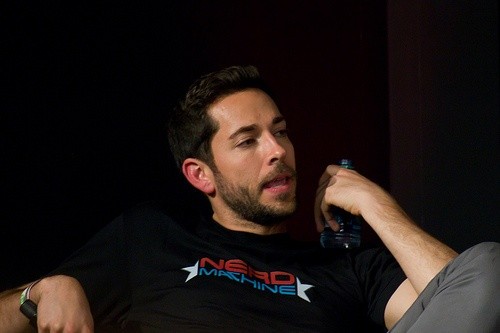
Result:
[0,64,500,333]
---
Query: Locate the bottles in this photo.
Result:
[320,159,361,250]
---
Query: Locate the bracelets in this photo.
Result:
[20,277,40,326]
